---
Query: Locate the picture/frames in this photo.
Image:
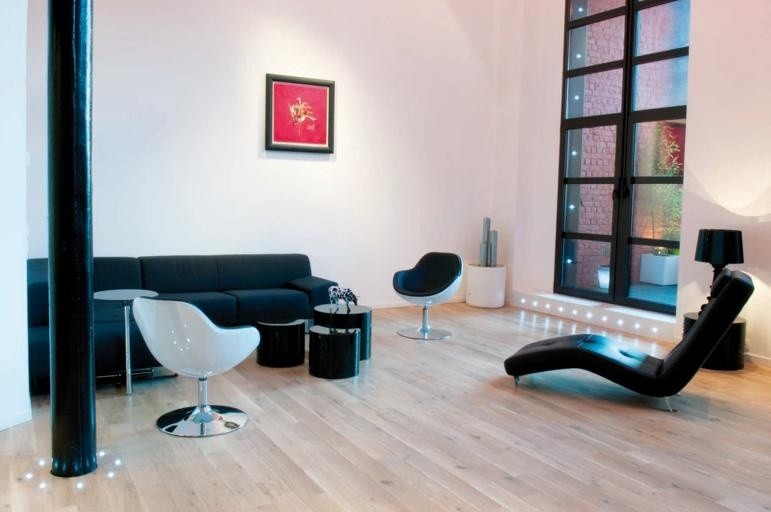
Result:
[266,73,336,154]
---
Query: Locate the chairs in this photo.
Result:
[504,267,753,412]
[393,253,462,341]
[132,297,261,438]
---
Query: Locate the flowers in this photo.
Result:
[328,286,358,306]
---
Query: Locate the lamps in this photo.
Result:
[694,230,744,316]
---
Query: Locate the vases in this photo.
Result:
[337,300,347,307]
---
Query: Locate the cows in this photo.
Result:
[328,286,358,314]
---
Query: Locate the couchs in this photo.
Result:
[27,253,339,397]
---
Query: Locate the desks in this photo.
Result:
[684,311,746,372]
[93,288,159,395]
[639,253,680,287]
